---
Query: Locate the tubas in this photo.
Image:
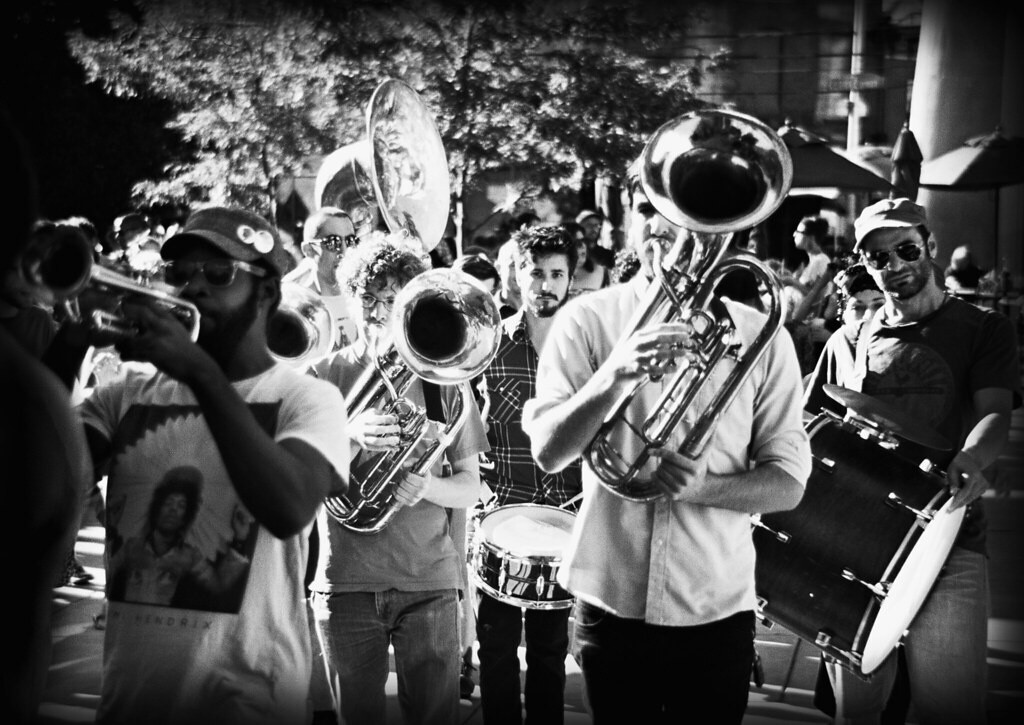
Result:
[314,267,503,535]
[583,108,796,500]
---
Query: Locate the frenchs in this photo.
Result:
[260,79,452,368]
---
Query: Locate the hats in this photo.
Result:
[851,196,928,254]
[575,209,598,225]
[160,206,284,278]
[111,212,147,251]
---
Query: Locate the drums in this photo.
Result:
[474,501,578,609]
[739,409,969,675]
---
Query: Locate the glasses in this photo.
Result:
[163,257,264,291]
[307,233,357,250]
[358,290,395,311]
[574,237,588,250]
[867,239,922,271]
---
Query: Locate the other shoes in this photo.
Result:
[454,650,477,699]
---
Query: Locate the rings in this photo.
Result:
[133,328,139,337]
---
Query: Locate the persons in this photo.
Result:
[0,208,1024,725]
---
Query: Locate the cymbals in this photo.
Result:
[821,382,955,454]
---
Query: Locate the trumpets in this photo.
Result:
[15,224,200,352]
